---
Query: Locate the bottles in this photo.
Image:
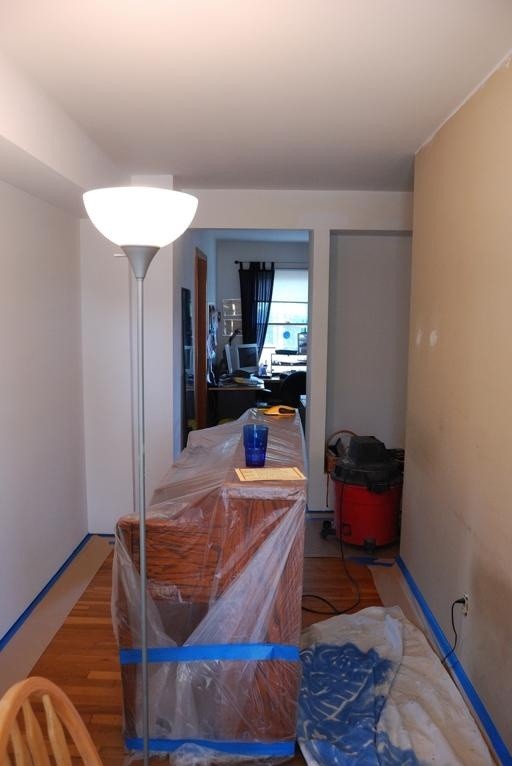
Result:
[259,360,268,374]
[298,327,307,354]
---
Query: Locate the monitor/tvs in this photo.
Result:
[230,344,259,373]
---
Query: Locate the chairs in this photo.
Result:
[252,371,306,432]
[0,676,109,766]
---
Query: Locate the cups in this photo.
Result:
[243,424,268,467]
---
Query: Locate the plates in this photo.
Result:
[274,350,297,355]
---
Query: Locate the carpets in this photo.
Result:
[299,514,391,560]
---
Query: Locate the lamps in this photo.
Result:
[81,185,200,766]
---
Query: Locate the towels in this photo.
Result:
[295,603,493,766]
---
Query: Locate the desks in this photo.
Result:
[207,371,282,428]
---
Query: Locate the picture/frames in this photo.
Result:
[222,299,243,316]
[221,319,243,336]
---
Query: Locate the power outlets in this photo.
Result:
[460,592,468,617]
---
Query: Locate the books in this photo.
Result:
[216,373,263,387]
